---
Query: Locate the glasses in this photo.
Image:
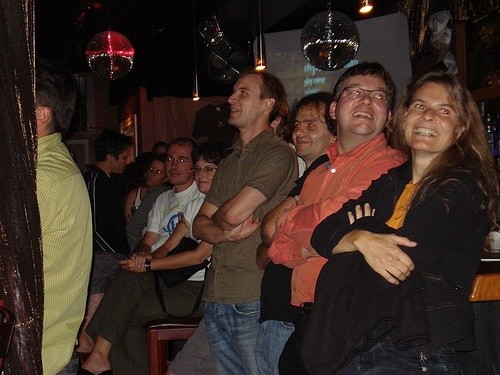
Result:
[164,155,192,163]
[148,167,164,174]
[336,87,392,105]
[190,165,217,173]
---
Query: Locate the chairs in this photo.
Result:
[144,316,203,375]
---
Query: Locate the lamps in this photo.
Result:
[191,66,201,102]
[358,0,374,17]
[252,0,267,72]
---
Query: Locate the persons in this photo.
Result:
[35,55,94,375]
[279,70,500,375]
[74,92,408,375]
[257,91,335,375]
[267,61,408,375]
[192,69,299,375]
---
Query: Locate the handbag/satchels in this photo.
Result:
[152,237,210,321]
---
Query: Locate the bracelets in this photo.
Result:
[145,259,151,272]
